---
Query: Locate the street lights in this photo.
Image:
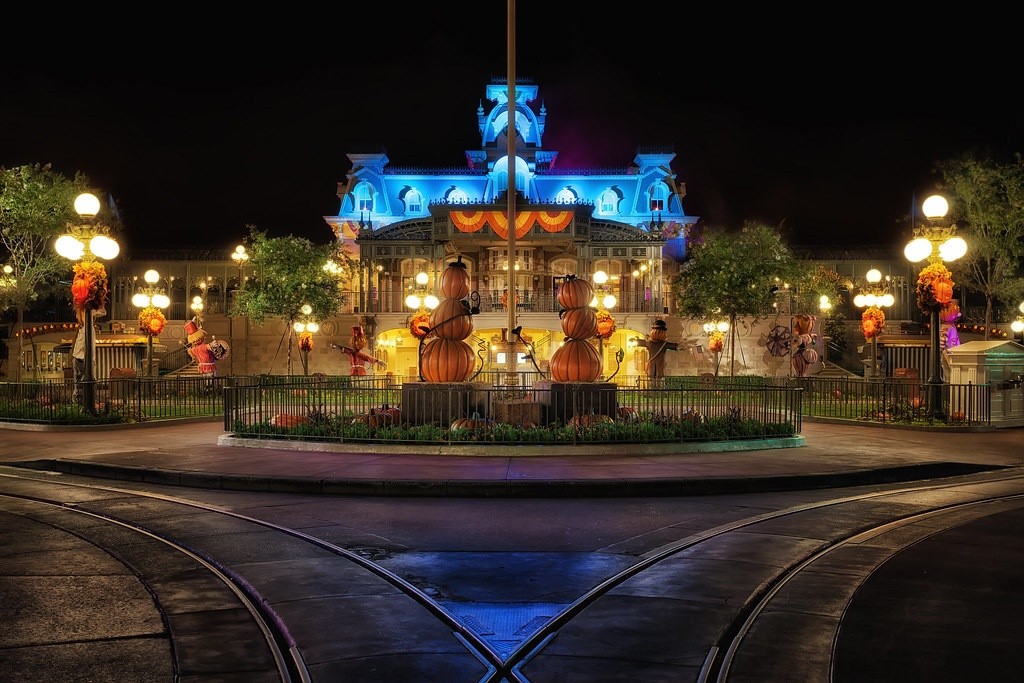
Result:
[232,243,248,311]
[132,268,172,398]
[703,306,730,377]
[853,267,895,399]
[293,304,319,377]
[587,270,617,381]
[903,193,968,418]
[52,192,122,418]
[190,294,205,330]
[404,271,440,355]
